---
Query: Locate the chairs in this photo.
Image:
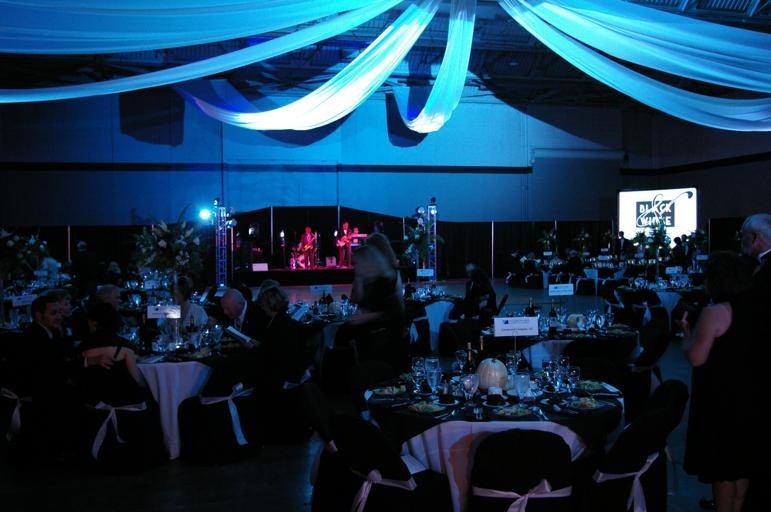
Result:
[78,366,153,509]
[465,427,583,512]
[578,416,658,511]
[640,379,691,450]
[192,364,259,467]
[521,259,544,290]
[629,288,670,338]
[405,298,431,360]
[0,374,54,469]
[310,408,427,512]
[503,257,524,290]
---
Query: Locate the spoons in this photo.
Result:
[441,409,458,422]
[479,408,483,420]
[533,412,543,423]
[474,408,479,420]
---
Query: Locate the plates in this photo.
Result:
[373,388,400,395]
[481,394,508,399]
[575,380,604,391]
[432,398,459,405]
[506,387,543,398]
[483,400,510,407]
[542,386,566,394]
[411,405,447,412]
[491,409,533,419]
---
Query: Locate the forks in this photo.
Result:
[434,409,449,418]
[538,409,549,421]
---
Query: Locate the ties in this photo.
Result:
[235,317,242,331]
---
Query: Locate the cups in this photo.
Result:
[556,304,567,322]
[411,357,425,371]
[425,357,440,371]
[461,374,479,401]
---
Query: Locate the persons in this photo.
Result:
[333,245,402,345]
[527,252,545,267]
[171,276,208,336]
[353,226,361,245]
[81,309,148,390]
[565,250,582,274]
[300,226,317,267]
[337,221,353,267]
[699,213,771,511]
[52,290,73,326]
[467,269,495,305]
[298,383,337,453]
[222,288,266,346]
[669,237,686,266]
[260,287,291,318]
[14,295,115,397]
[616,232,630,255]
[681,235,688,251]
[682,252,771,512]
[367,233,406,319]
[98,284,121,309]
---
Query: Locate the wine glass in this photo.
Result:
[606,313,615,327]
[595,315,605,333]
[566,366,580,396]
[457,350,467,369]
[514,372,530,409]
[410,372,425,400]
[427,368,442,399]
[530,378,540,400]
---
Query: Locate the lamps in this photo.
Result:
[416,205,425,215]
[194,206,215,222]
